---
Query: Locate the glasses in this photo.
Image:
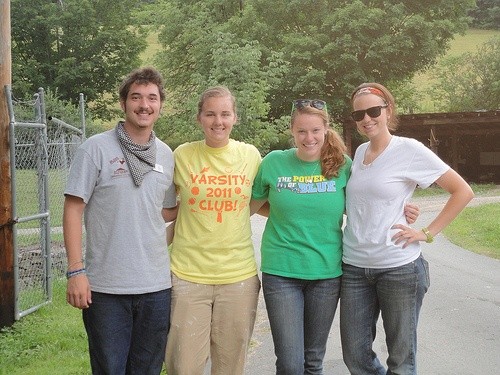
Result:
[292,99,326,114]
[350,105,389,121]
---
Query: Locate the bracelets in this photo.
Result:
[66,261,86,279]
[422,227,433,242]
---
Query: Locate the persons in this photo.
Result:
[62,70,179,375]
[167,86,270,375]
[166,100,419,375]
[340,82,474,375]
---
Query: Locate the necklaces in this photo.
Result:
[295,150,320,183]
